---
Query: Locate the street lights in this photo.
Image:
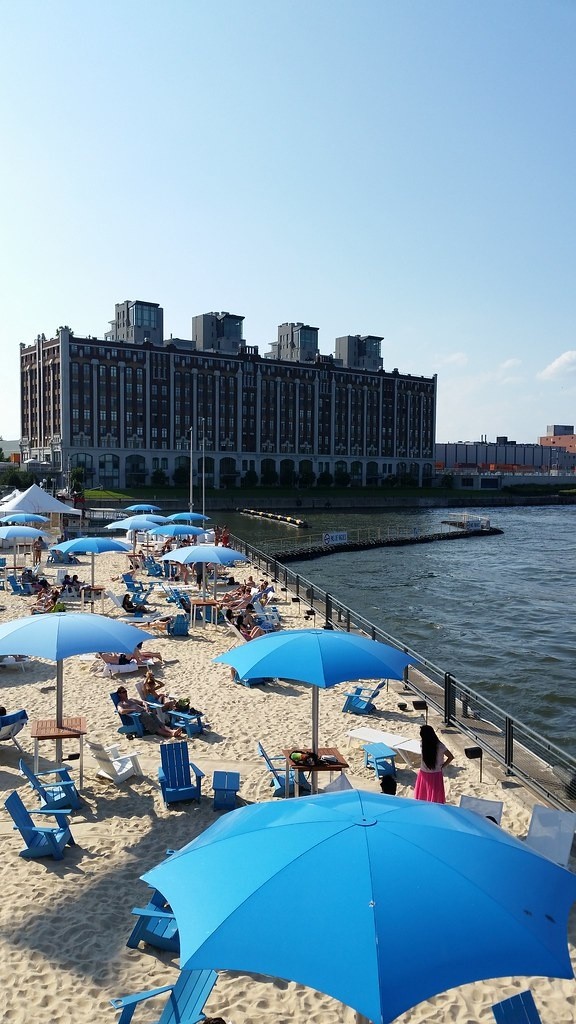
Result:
[200,417,205,527]
[189,425,195,512]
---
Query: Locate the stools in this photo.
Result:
[362,743,396,778]
[214,770,240,810]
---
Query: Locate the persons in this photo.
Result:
[35,537,44,564]
[162,535,197,555]
[123,594,157,613]
[116,686,182,737]
[136,617,172,634]
[215,525,230,546]
[413,725,454,804]
[139,550,144,559]
[142,673,164,700]
[220,576,269,640]
[22,570,82,613]
[101,647,149,666]
[180,598,191,608]
[168,563,209,590]
[131,651,162,661]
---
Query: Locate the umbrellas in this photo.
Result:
[0,527,50,577]
[126,505,162,511]
[105,512,212,536]
[0,612,157,783]
[142,789,576,1024]
[0,513,52,526]
[161,544,247,628]
[212,628,416,796]
[48,537,134,613]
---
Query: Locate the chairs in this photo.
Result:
[96,651,150,679]
[0,709,29,755]
[47,549,77,565]
[134,681,177,720]
[492,991,541,1024]
[84,740,144,783]
[111,692,156,738]
[0,553,87,615]
[146,555,178,577]
[166,613,191,637]
[126,848,186,952]
[109,971,218,1024]
[256,742,312,796]
[346,727,423,768]
[459,795,576,869]
[4,792,75,860]
[341,680,385,714]
[218,586,281,652]
[107,591,163,618]
[18,759,83,812]
[158,741,204,805]
[123,573,154,592]
[162,585,192,613]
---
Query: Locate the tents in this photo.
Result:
[3,483,82,536]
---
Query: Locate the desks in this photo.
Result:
[17,543,32,561]
[126,554,143,571]
[30,718,87,791]
[191,599,217,630]
[281,748,348,798]
[4,566,25,583]
[167,709,205,739]
